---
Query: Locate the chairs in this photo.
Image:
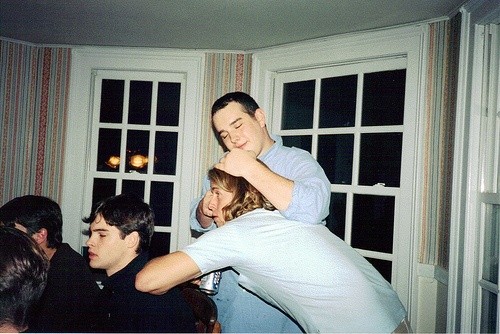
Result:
[179,284,218,334]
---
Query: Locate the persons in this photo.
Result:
[188,91,332,334]
[81,194,197,333]
[134,158,413,334]
[0,195,101,333]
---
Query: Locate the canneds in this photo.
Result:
[198,272,222,295]
